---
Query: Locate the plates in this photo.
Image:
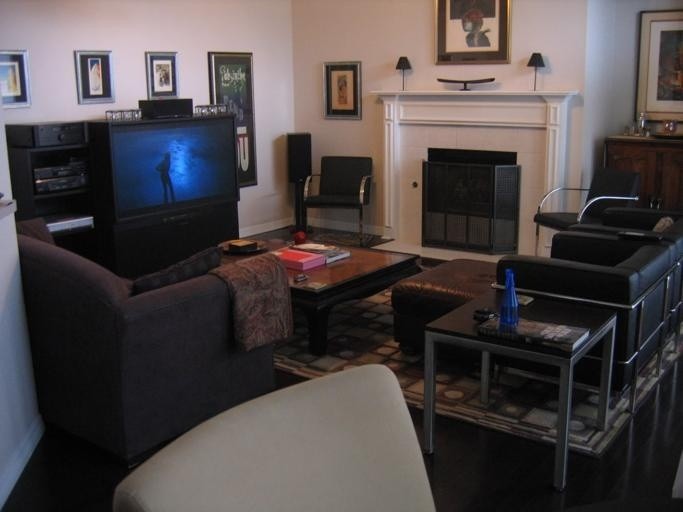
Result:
[217,238,268,255]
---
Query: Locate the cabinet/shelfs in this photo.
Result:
[603,135,683,211]
[3,119,98,220]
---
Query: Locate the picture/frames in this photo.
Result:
[75,49,114,104]
[634,10,683,121]
[434,0,517,65]
[209,52,257,187]
[323,62,362,120]
[0,49,31,109]
[146,52,179,101]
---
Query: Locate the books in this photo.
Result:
[477,316,590,353]
[272,242,351,272]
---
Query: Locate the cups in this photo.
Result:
[663,119,677,136]
[286,239,295,248]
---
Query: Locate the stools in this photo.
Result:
[391,259,497,355]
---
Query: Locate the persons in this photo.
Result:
[90,60,100,82]
[338,80,347,104]
[461,8,490,47]
[155,152,176,208]
[157,63,168,84]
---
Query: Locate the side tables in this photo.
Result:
[422,291,616,494]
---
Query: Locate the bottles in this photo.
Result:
[500,267,520,327]
[623,112,651,139]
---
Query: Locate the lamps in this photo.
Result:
[396,57,412,90]
[526,53,545,91]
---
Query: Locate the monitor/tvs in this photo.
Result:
[97,112,240,233]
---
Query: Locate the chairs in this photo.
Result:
[112,363,439,512]
[492,229,678,413]
[14,217,291,459]
[533,167,640,256]
[302,155,374,247]
[568,207,683,352]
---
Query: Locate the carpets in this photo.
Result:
[273,281,683,459]
